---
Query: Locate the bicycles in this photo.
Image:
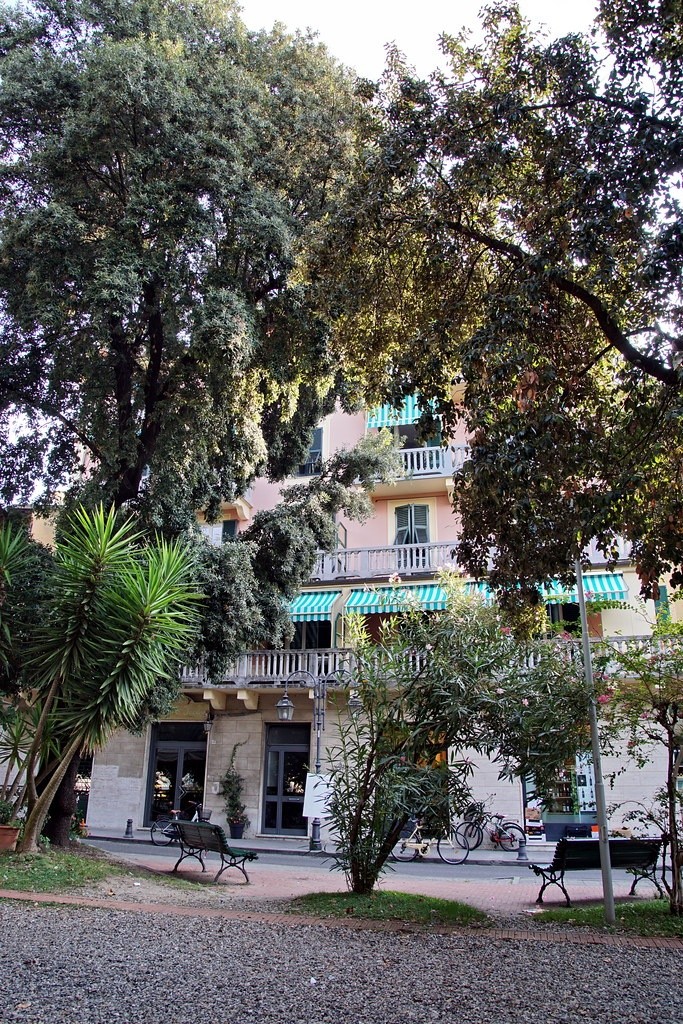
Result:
[391,822,470,865]
[150,801,212,846]
[455,794,527,852]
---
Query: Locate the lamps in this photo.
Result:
[203,712,215,734]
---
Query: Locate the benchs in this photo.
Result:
[167,820,260,885]
[526,836,664,908]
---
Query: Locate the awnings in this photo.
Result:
[282,591,342,622]
[344,570,629,615]
[368,393,439,428]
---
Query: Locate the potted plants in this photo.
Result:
[216,770,251,839]
[0,799,20,852]
